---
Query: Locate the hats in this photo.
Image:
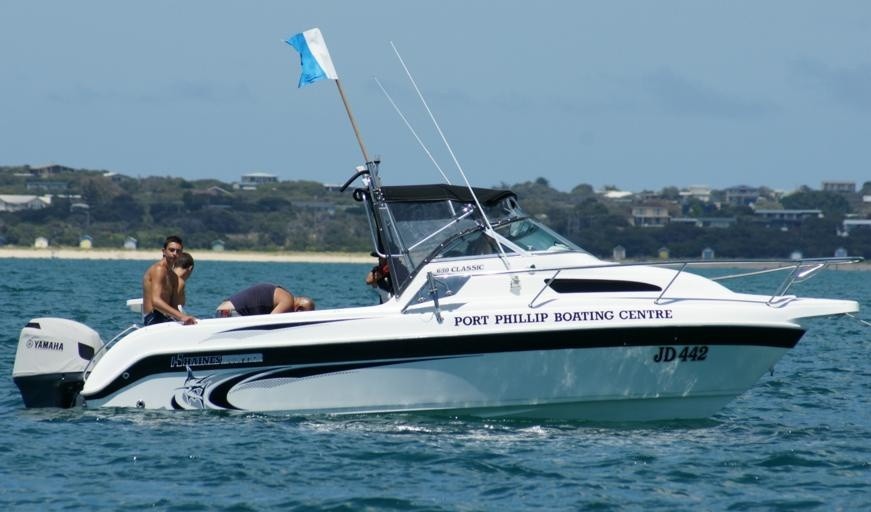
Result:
[370,242,400,258]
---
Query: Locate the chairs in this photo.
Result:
[466,239,493,255]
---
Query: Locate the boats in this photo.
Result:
[12,41,864,420]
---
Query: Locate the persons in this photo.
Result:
[174,252,195,307]
[216,284,315,317]
[143,236,198,325]
[366,242,409,302]
